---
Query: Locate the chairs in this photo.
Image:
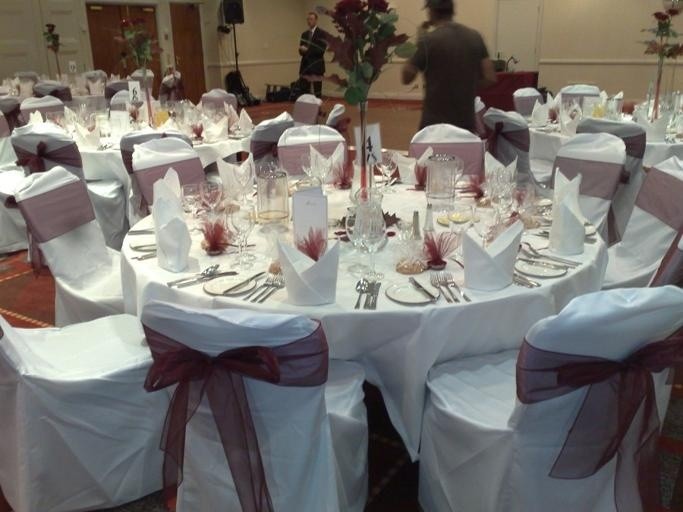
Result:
[250,110,295,161]
[512,87,543,118]
[292,94,324,126]
[32,82,73,100]
[276,125,348,173]
[10,71,39,83]
[83,70,109,90]
[409,123,486,187]
[154,70,182,112]
[130,138,207,214]
[576,119,648,246]
[0,169,28,254]
[135,299,370,512]
[0,95,21,125]
[119,133,193,228]
[202,88,238,118]
[10,121,128,251]
[550,132,627,200]
[416,284,682,510]
[0,315,175,512]
[105,81,128,107]
[601,155,683,290]
[108,89,129,119]
[473,95,487,138]
[324,104,346,128]
[482,107,553,196]
[0,109,17,171]
[18,95,66,120]
[15,165,125,329]
[130,69,154,101]
[558,83,601,119]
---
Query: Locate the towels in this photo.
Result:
[462,219,524,291]
[151,197,192,273]
[276,236,340,306]
[393,145,433,184]
[217,153,256,191]
[531,99,550,127]
[88,78,101,95]
[558,106,579,136]
[548,167,584,255]
[29,110,43,126]
[74,121,100,146]
[636,110,667,143]
[484,151,519,178]
[204,117,228,143]
[309,142,345,181]
[239,109,253,134]
[546,92,561,106]
[153,168,180,215]
[599,90,624,104]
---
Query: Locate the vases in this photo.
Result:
[355,101,367,207]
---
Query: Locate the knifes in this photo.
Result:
[518,257,568,271]
[176,270,239,289]
[363,278,377,309]
[408,275,436,302]
[368,282,382,310]
[221,269,265,296]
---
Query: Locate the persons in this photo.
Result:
[298,11,329,112]
[400,1,497,133]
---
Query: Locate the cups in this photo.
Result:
[180,182,206,237]
[384,150,401,193]
[316,153,334,194]
[343,205,371,275]
[252,158,279,179]
[232,161,255,209]
[258,169,290,234]
[239,211,258,262]
[423,154,465,214]
[447,203,472,234]
[436,208,453,230]
[514,181,536,218]
[584,225,596,236]
[473,205,501,252]
[375,152,391,192]
[353,203,387,280]
[397,220,415,260]
[491,183,515,227]
[200,180,223,222]
[298,153,319,188]
[226,209,253,270]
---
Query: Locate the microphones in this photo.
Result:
[496,51,501,60]
[505,55,519,72]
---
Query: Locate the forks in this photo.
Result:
[251,270,284,304]
[521,247,578,267]
[522,241,583,264]
[257,279,285,304]
[429,271,453,304]
[437,272,461,303]
[443,271,473,303]
[242,274,270,302]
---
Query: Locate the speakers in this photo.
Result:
[222,0,244,24]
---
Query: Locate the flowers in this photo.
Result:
[42,23,65,82]
[635,0,683,121]
[102,17,164,128]
[299,1,411,197]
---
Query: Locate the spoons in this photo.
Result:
[167,262,219,287]
[354,277,369,310]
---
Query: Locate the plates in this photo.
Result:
[514,256,568,280]
[385,283,442,305]
[202,274,256,297]
